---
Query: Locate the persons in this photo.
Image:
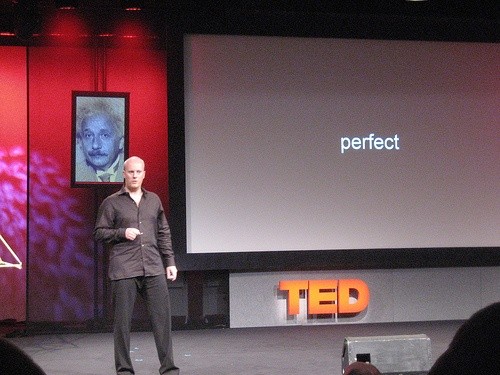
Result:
[75,101,125,183]
[94,157,179,375]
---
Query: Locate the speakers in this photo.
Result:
[341,334,433,375]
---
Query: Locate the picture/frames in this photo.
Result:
[71,89,130,189]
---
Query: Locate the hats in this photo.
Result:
[344,362,381,375]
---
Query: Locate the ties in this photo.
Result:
[99,173,111,182]
